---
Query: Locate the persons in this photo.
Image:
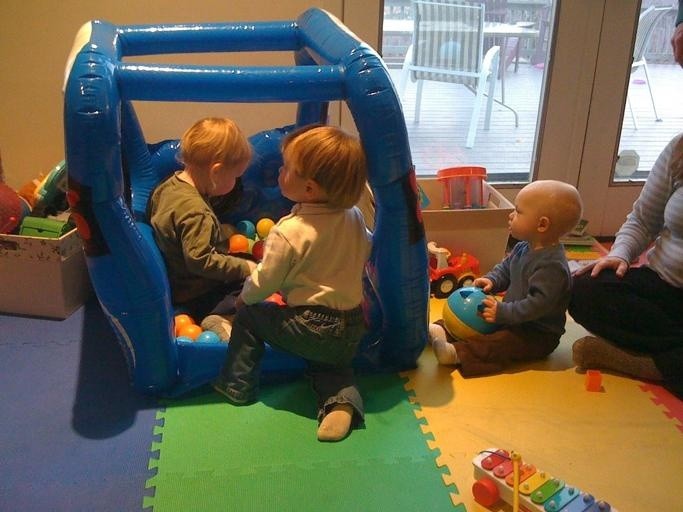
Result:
[426,180,583,375]
[216,127,373,442]
[147,117,258,343]
[567,128,683,399]
[669,0,683,68]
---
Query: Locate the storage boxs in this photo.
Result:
[0,226,93,320]
[361,178,517,277]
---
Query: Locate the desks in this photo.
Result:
[383,19,540,128]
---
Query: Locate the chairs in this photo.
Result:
[627,4,674,131]
[397,1,500,149]
